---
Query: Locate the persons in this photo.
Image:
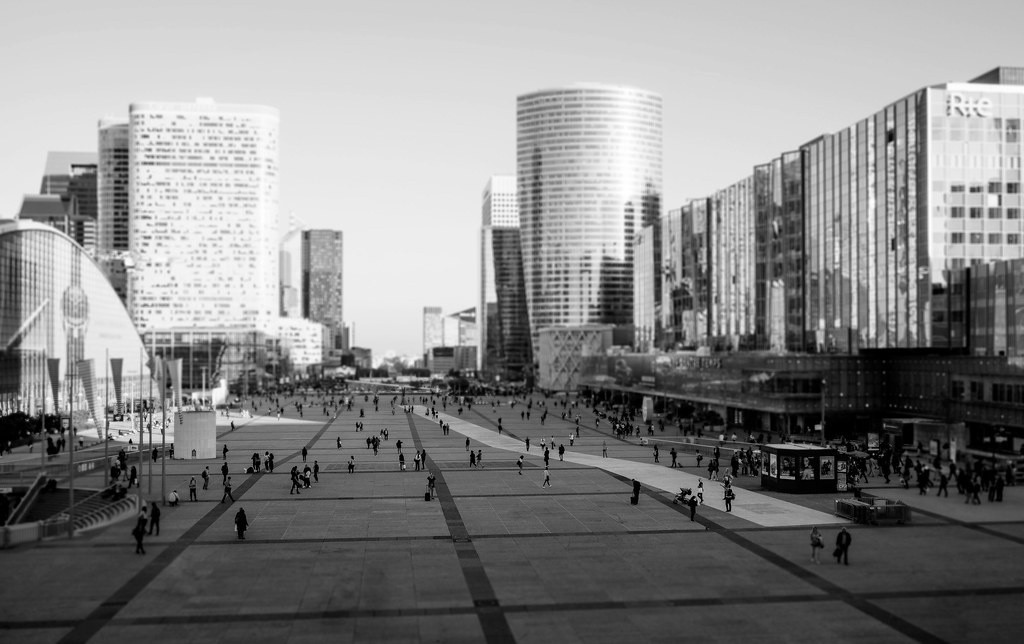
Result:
[2,372,1024,506]
[688,496,698,521]
[234,507,248,539]
[722,484,735,512]
[835,527,851,565]
[148,501,160,536]
[132,506,148,555]
[810,526,824,565]
